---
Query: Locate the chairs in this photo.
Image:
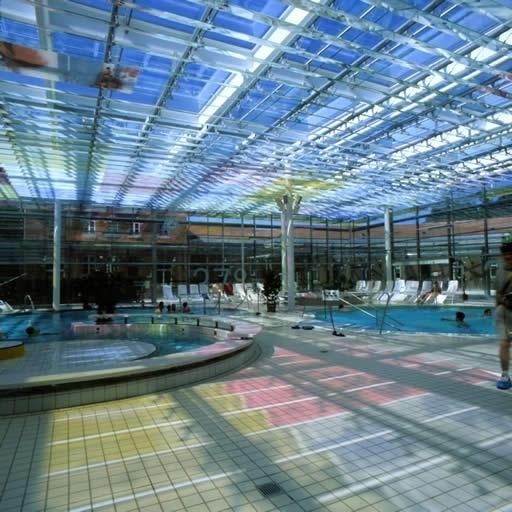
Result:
[343,278,459,306]
[162,283,267,305]
[0,300,30,314]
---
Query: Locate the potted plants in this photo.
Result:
[261,269,281,312]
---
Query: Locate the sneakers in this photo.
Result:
[497,374,511,390]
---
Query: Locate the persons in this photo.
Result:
[26,326,41,336]
[181,302,191,312]
[224,278,235,302]
[154,301,164,313]
[416,281,442,304]
[440,312,464,324]
[494,242,511,389]
[167,305,171,312]
[482,308,492,316]
[172,303,177,312]
